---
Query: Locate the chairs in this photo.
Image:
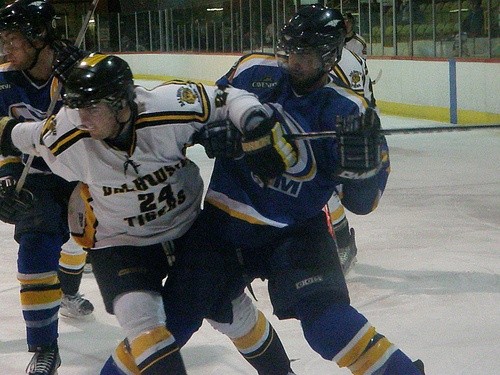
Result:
[370,0,500,41]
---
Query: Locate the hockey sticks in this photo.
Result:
[13,1,99,196]
[285,124,498,139]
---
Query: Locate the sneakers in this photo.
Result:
[335,227,358,278]
[25,342,61,375]
[60,292,94,319]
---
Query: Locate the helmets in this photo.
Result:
[0,0,59,44]
[60,52,137,131]
[341,10,355,23]
[274,3,347,89]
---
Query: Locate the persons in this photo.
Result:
[101,4,425,375]
[392,0,424,24]
[322,11,376,274]
[0,51,299,375]
[0,0,94,375]
[121,13,280,53]
[461,0,484,37]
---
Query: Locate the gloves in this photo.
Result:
[233,134,300,200]
[335,107,383,180]
